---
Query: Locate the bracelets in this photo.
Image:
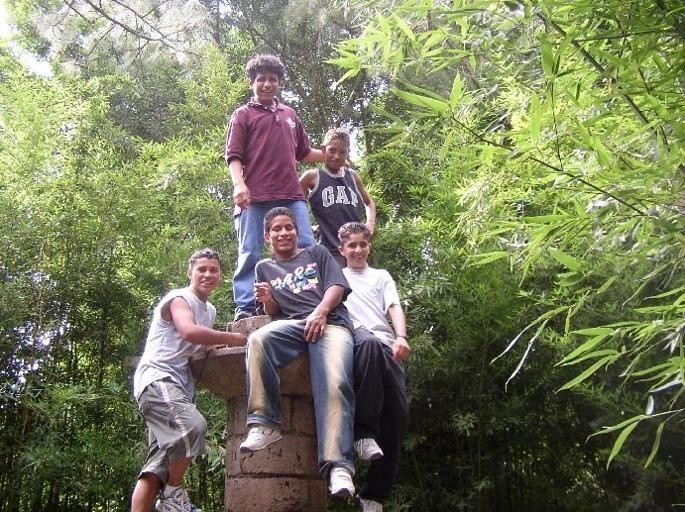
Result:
[396,335,410,342]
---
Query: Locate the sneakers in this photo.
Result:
[353,438,385,461]
[355,494,383,512]
[328,466,355,497]
[240,423,283,452]
[154,487,203,512]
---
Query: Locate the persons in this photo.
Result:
[337,221,411,512]
[239,206,357,499]
[300,128,377,267]
[130,249,246,512]
[225,54,350,321]
